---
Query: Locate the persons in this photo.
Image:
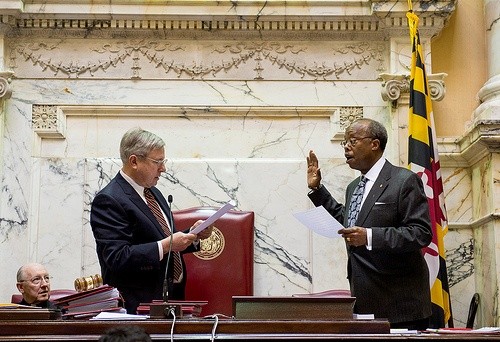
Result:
[90,127,213,315]
[307,118,432,329]
[16,264,66,314]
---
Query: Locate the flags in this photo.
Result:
[406,12,454,328]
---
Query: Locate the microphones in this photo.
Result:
[150,195,181,319]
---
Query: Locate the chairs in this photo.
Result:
[172,205,255,315]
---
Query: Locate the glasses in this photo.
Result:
[19,274,54,285]
[144,156,168,168]
[340,136,372,147]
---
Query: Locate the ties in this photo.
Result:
[143,187,183,281]
[347,176,368,228]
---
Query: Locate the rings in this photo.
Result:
[347,237,350,242]
[309,164,312,166]
